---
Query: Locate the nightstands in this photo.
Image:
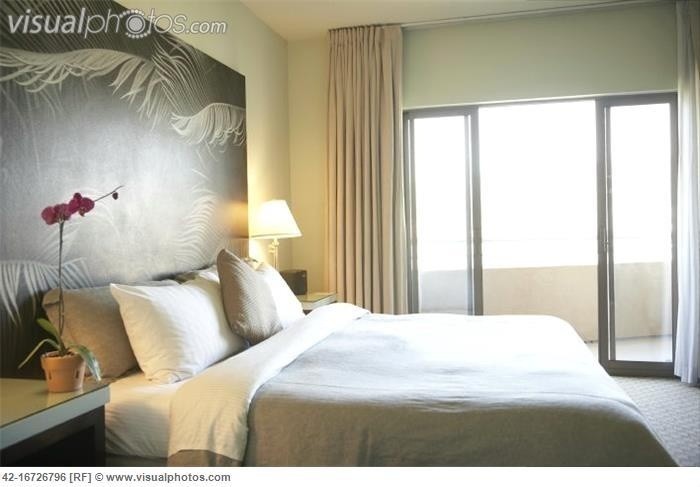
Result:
[296,291,337,315]
[0,377,111,466]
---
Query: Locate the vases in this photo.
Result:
[40,350,86,393]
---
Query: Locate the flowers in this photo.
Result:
[19,184,124,381]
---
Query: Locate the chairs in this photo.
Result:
[41,246,306,385]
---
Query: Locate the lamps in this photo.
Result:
[250,198,303,269]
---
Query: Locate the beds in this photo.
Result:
[101,301,679,466]
